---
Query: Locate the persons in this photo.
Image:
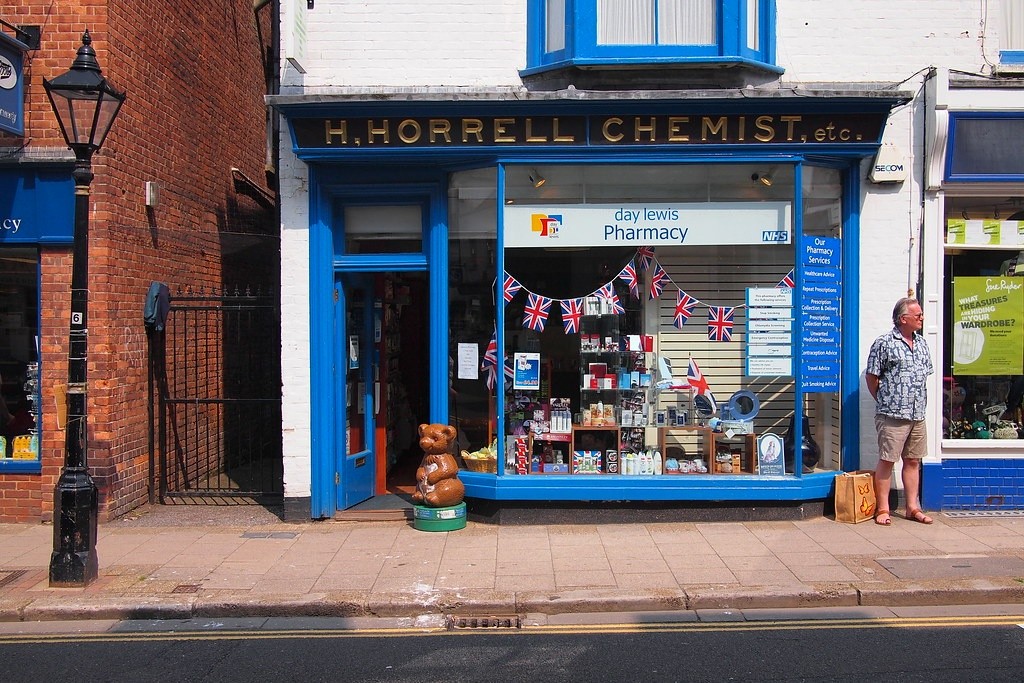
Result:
[865,297,935,526]
[419,326,460,438]
[450,312,489,460]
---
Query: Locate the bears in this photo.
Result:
[411,424,464,508]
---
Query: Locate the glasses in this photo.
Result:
[901,312,923,318]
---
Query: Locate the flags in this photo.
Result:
[649,263,671,303]
[707,307,735,341]
[561,300,584,335]
[776,271,795,287]
[674,290,698,330]
[481,326,514,391]
[492,270,520,309]
[687,356,718,414]
[595,284,625,315]
[620,259,640,301]
[522,294,552,332]
[636,245,655,271]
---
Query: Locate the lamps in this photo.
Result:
[146,182,159,208]
[527,166,546,189]
[962,200,1024,220]
[761,166,782,187]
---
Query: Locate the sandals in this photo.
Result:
[875,510,891,526]
[906,509,933,524]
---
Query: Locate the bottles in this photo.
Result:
[597,400,603,418]
[620,450,662,475]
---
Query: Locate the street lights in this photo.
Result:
[42,28,129,584]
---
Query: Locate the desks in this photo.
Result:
[527,422,755,476]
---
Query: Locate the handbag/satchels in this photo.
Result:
[835,469,878,524]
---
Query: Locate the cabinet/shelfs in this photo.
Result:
[374,276,433,475]
